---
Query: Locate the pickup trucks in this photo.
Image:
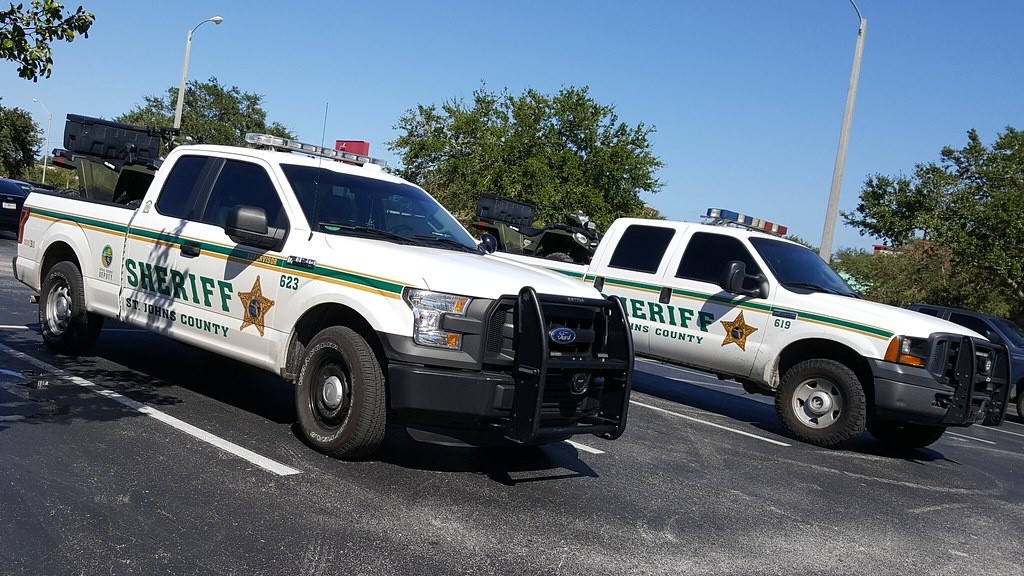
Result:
[12,132,634,459]
[495,207,1013,448]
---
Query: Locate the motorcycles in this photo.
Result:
[472,193,604,264]
[52,114,199,207]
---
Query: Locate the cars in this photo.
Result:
[907,303,1024,422]
[0,178,28,232]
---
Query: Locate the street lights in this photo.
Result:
[174,16,223,128]
[32,98,52,183]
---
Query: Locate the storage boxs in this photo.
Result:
[476,193,536,227]
[64,114,160,159]
